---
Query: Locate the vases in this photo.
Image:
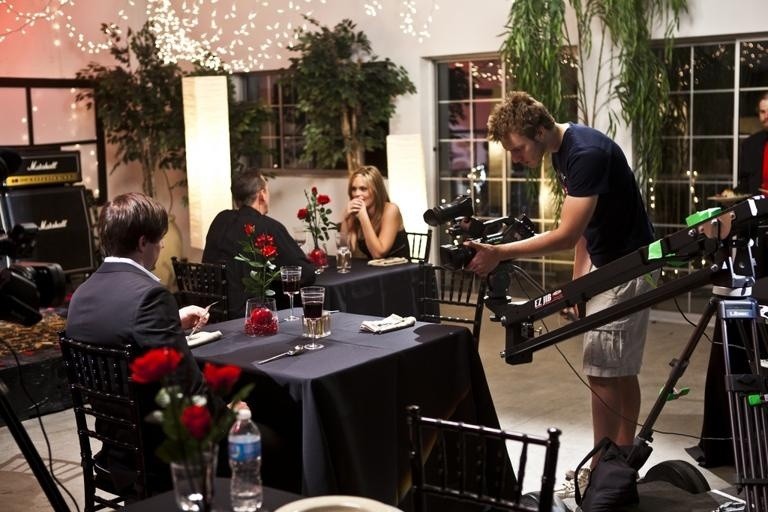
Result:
[308,243,329,269]
[169,442,220,511]
[245,298,279,337]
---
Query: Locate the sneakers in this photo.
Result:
[556,468,591,500]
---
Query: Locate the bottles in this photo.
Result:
[227,410,265,512]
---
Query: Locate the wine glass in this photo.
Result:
[278,264,303,322]
[333,228,352,273]
[299,286,328,352]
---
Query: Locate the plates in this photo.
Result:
[275,494,404,511]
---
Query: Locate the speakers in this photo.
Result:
[0,185,95,275]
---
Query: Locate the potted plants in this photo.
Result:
[75,15,275,291]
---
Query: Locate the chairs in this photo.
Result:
[57,329,145,512]
[171,255,230,323]
[415,260,487,353]
[404,229,432,265]
[405,404,562,512]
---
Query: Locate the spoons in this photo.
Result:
[257,343,302,365]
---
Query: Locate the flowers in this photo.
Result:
[128,347,257,511]
[297,187,338,249]
[233,224,282,304]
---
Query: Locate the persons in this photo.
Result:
[65,192,229,492]
[716,92,768,198]
[464,92,662,497]
[339,166,410,264]
[202,168,315,320]
